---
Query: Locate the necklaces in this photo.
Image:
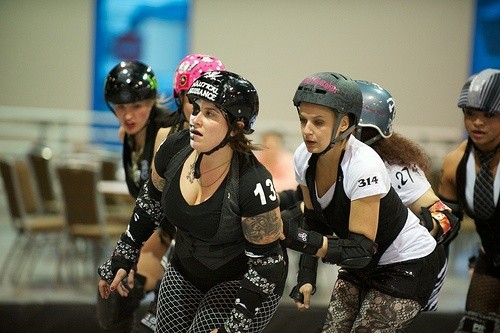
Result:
[186,152,230,187]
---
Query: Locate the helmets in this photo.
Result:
[103,60,159,105]
[294,73,364,125]
[173,54,225,99]
[457,68,500,115]
[352,80,397,139]
[187,70,260,135]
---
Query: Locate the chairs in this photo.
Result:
[0,148,136,292]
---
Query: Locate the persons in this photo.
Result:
[256,68,500,333]
[98,71,289,333]
[105,54,225,333]
